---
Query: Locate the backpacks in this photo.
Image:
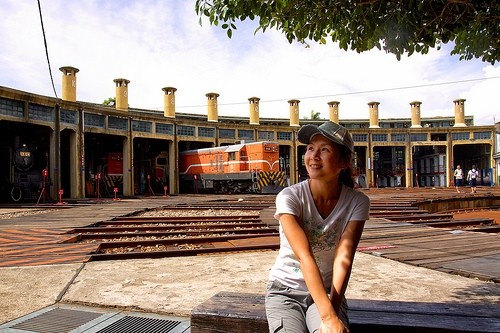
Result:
[471,173,477,179]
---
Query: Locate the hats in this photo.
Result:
[297,121,355,154]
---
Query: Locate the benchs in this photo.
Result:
[187,290,500,333]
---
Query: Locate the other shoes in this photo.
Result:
[471,190,476,193]
[457,190,464,193]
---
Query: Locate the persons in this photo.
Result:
[262,120,370,333]
[453,165,464,194]
[466,164,478,194]
